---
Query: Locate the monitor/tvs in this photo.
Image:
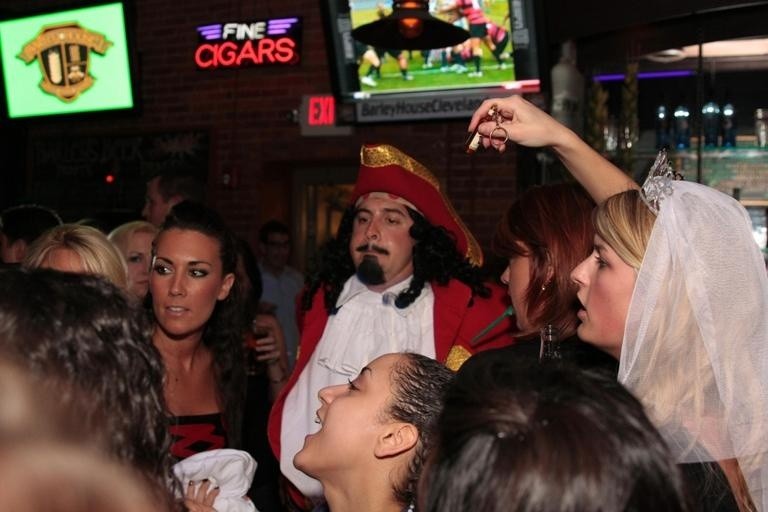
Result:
[326,1,544,125]
[1,0,135,120]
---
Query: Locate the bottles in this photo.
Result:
[755,107,768,148]
[702,101,720,151]
[722,103,738,151]
[657,106,670,151]
[537,323,565,362]
[674,105,690,152]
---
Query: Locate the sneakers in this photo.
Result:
[362,75,376,87]
[441,65,449,72]
[457,66,469,75]
[469,71,483,77]
[422,62,433,68]
[497,64,506,69]
[403,74,413,81]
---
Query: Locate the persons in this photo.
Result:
[21,223,129,291]
[145,198,266,463]
[0,267,174,512]
[0,205,63,261]
[106,219,159,305]
[468,94,768,512]
[266,144,520,512]
[500,181,596,341]
[349,1,509,86]
[294,352,456,512]
[393,355,690,512]
[255,218,306,374]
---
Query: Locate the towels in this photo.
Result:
[168,448,257,512]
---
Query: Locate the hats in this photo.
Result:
[350,141,485,268]
